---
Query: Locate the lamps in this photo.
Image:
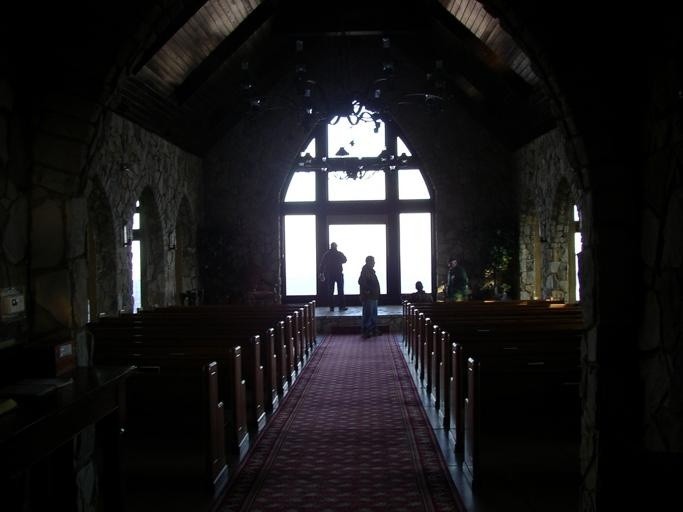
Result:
[244,30,448,182]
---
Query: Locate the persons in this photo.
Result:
[357,257,384,337]
[412,281,433,303]
[445,255,465,302]
[318,242,349,312]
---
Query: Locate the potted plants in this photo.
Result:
[469,231,513,300]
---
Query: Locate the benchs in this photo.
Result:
[397,292,581,496]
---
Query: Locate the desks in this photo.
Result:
[0,362,138,512]
